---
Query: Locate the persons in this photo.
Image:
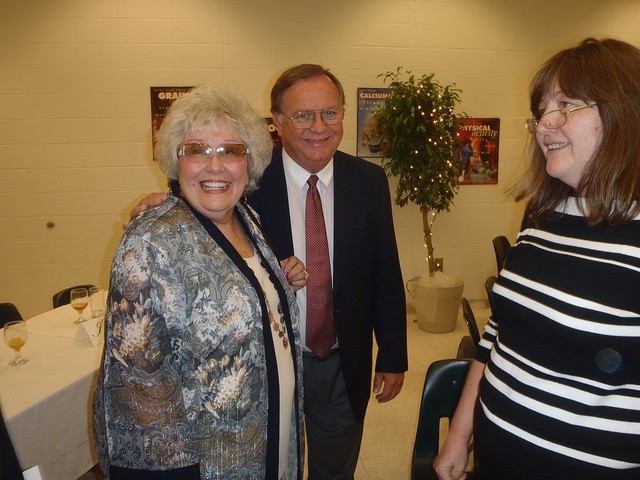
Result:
[431,36,639,480]
[92,83,310,480]
[459,139,474,165]
[121,63,409,480]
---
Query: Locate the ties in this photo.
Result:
[305,175,336,360]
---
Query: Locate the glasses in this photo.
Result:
[525,103,598,135]
[279,108,344,129]
[177,142,250,164]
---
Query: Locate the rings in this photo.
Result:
[303,269,309,282]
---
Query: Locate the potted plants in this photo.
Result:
[373,65,464,335]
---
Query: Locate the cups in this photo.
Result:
[89,286,105,318]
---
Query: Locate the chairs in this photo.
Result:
[457,290,485,358]
[52,282,97,308]
[411,358,483,480]
[484,274,510,319]
[0,302,24,327]
[492,231,513,270]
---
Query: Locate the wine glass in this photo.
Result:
[3,321,30,366]
[71,287,89,324]
